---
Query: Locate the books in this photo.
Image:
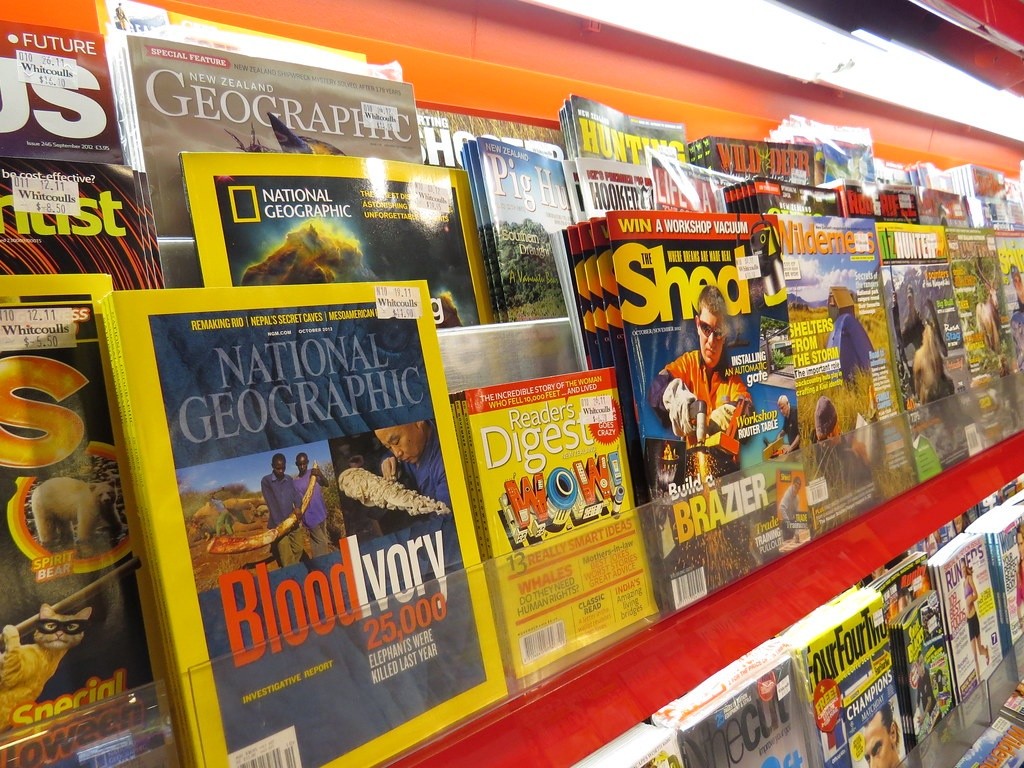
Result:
[0,1,1024,768]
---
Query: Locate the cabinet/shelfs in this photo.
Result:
[0,0,1023,768]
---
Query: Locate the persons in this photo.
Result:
[862,704,900,768]
[115,3,133,31]
[1016,524,1024,619]
[961,559,990,685]
[810,397,876,516]
[777,394,799,455]
[649,286,755,463]
[917,650,942,733]
[293,453,330,558]
[892,284,948,384]
[261,453,303,567]
[1010,265,1024,369]
[780,476,801,540]
[364,420,463,573]
